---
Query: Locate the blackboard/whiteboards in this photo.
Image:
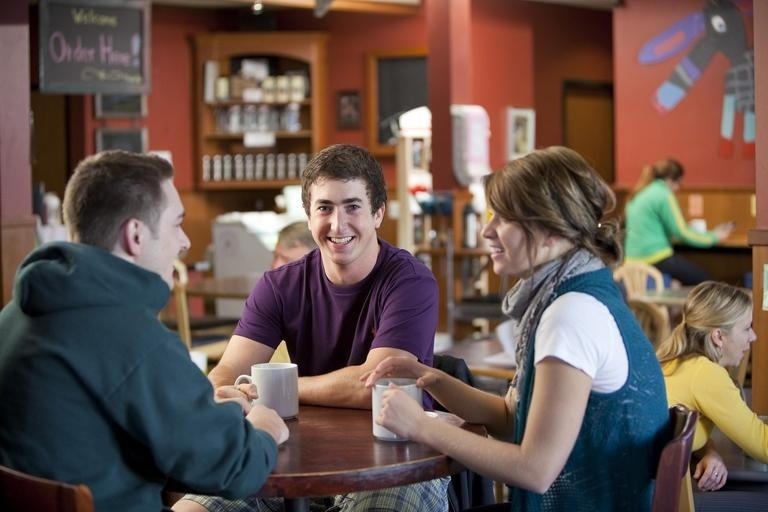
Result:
[40,1,153,96]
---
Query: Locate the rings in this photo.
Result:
[712,471,718,476]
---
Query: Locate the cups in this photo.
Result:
[371,376,423,443]
[235,363,301,422]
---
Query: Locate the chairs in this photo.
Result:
[624,301,666,350]
[156,280,240,361]
[612,263,664,293]
[652,404,698,512]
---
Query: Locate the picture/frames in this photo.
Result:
[336,89,362,130]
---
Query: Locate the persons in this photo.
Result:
[169,143,452,512]
[656,281,767,512]
[0,150,293,512]
[358,147,671,512]
[624,157,732,289]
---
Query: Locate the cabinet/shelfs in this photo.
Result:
[195,32,331,190]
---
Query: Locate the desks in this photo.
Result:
[165,399,488,510]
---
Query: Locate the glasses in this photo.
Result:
[675,178,681,186]
